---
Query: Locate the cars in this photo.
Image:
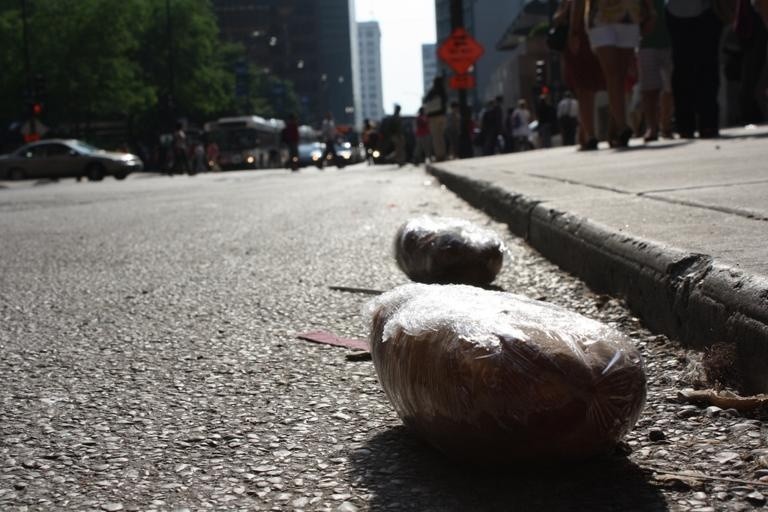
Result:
[287,115,426,172]
[0,133,145,186]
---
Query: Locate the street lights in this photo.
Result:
[241,25,356,125]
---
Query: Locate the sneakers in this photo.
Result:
[699,127,719,138]
[680,127,696,138]
[577,136,598,151]
[609,127,634,148]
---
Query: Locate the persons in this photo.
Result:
[549,0,767,152]
[284,112,300,170]
[384,105,406,166]
[156,122,220,176]
[415,76,548,162]
[363,120,377,165]
[314,111,345,169]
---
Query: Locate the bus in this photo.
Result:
[201,110,293,173]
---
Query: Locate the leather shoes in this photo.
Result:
[661,127,674,139]
[645,125,660,142]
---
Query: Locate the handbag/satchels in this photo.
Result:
[548,1,571,52]
